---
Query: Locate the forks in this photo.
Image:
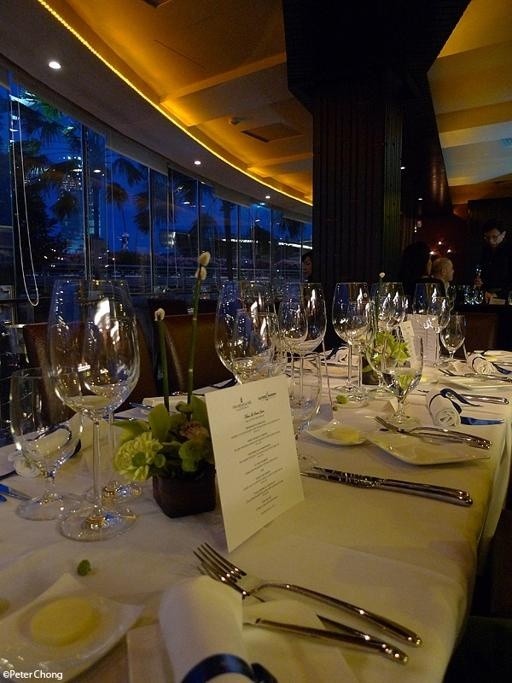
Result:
[190,541,429,645]
[169,389,206,397]
[376,415,492,449]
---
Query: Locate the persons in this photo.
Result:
[301,250,311,284]
[432,256,455,299]
[395,241,446,310]
[469,222,512,297]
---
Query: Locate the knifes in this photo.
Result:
[128,402,181,415]
[315,613,409,664]
[245,613,393,657]
[299,471,473,506]
[312,465,471,500]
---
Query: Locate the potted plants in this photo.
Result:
[114,251,217,518]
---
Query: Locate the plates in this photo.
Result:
[0,569,145,676]
[363,429,493,468]
[301,416,376,446]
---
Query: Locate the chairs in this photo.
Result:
[23,318,158,424]
[153,313,232,393]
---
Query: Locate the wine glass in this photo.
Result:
[212,278,511,429]
[1,280,144,542]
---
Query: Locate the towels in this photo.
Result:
[426,387,502,430]
[336,347,349,363]
[468,353,511,375]
[14,411,108,479]
[158,575,252,683]
